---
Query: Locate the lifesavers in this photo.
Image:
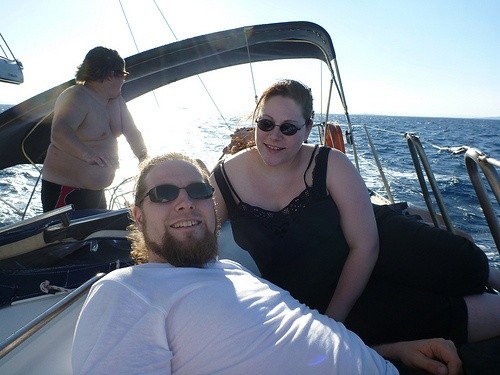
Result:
[325,121,346,154]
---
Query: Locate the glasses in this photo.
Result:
[138,183,215,205]
[121,71,130,81]
[255,118,306,136]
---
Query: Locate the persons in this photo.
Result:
[72,152,463,375]
[208,79,499,374]
[41,47,148,214]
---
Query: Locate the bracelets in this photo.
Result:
[137,153,148,162]
